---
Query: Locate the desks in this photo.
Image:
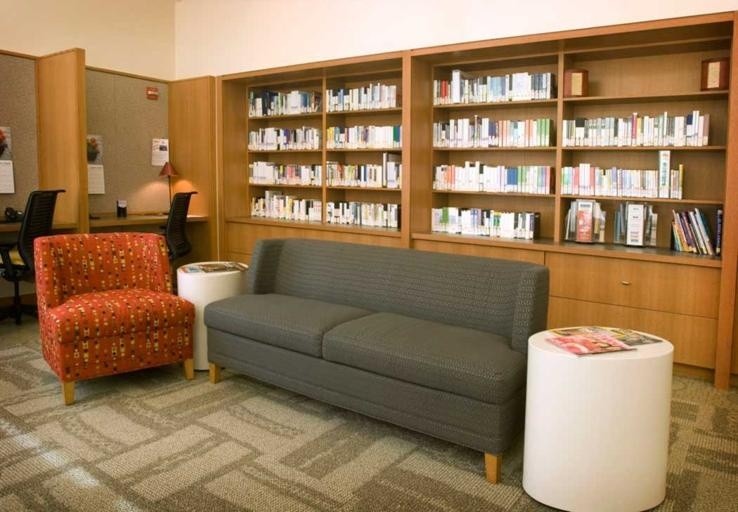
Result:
[1,217,78,234]
[88,209,212,230]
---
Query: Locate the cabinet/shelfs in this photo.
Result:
[216,52,410,239]
[410,238,721,372]
[406,8,736,270]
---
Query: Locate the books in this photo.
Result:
[551,325,612,338]
[545,334,635,356]
[562,110,711,146]
[430,206,541,241]
[198,262,246,272]
[179,264,205,275]
[248,88,324,149]
[560,150,684,200]
[433,115,554,148]
[325,82,402,148]
[433,69,555,105]
[248,161,322,222]
[326,152,402,228]
[432,160,555,194]
[608,328,662,346]
[564,198,723,256]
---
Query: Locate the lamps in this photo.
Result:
[156,161,182,214]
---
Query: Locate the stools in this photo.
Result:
[173,255,250,376]
[521,323,676,512]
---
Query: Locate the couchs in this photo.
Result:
[202,233,553,490]
[31,229,197,408]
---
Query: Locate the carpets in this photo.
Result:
[0,338,737,512]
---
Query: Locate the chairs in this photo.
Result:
[152,189,199,296]
[1,185,67,326]
